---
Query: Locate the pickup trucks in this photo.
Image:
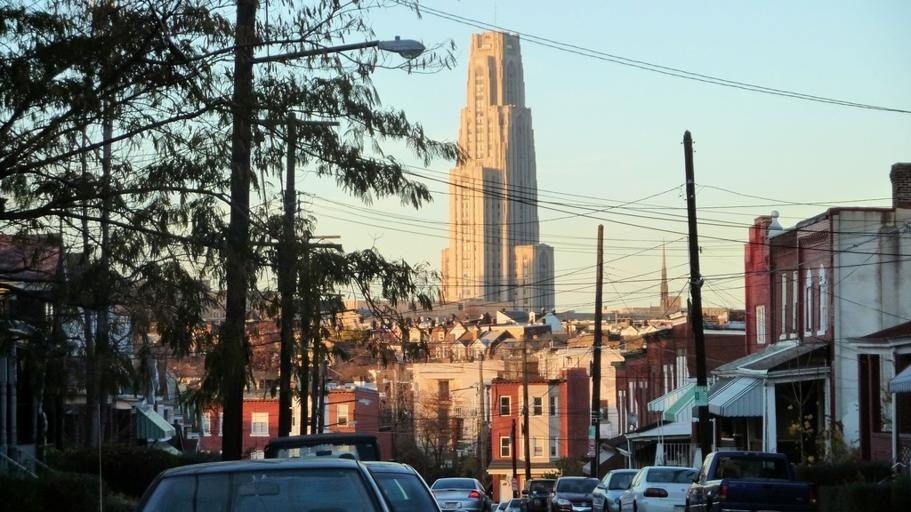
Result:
[685,450,819,512]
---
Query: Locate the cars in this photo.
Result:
[492,466,700,512]
